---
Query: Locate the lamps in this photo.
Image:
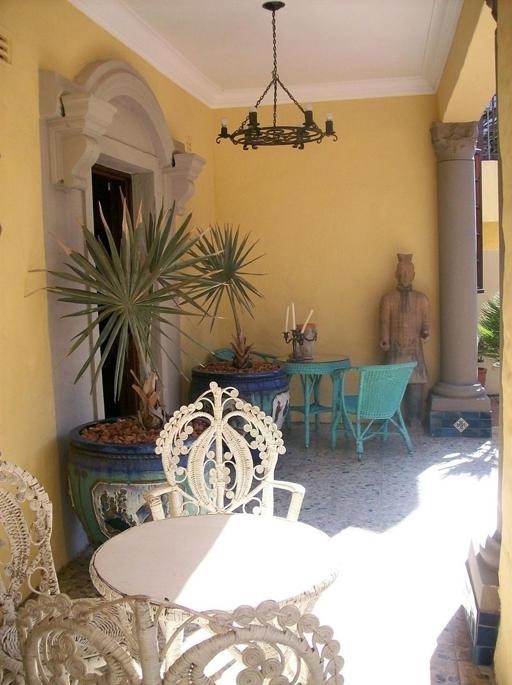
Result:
[214,0,341,152]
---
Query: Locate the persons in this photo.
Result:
[377,252,432,430]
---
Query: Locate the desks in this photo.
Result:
[272,352,350,450]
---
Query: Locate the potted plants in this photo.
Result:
[178,224,289,463]
[23,183,232,547]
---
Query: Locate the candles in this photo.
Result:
[284,302,315,334]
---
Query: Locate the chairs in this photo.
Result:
[331,362,420,460]
[0,379,346,685]
